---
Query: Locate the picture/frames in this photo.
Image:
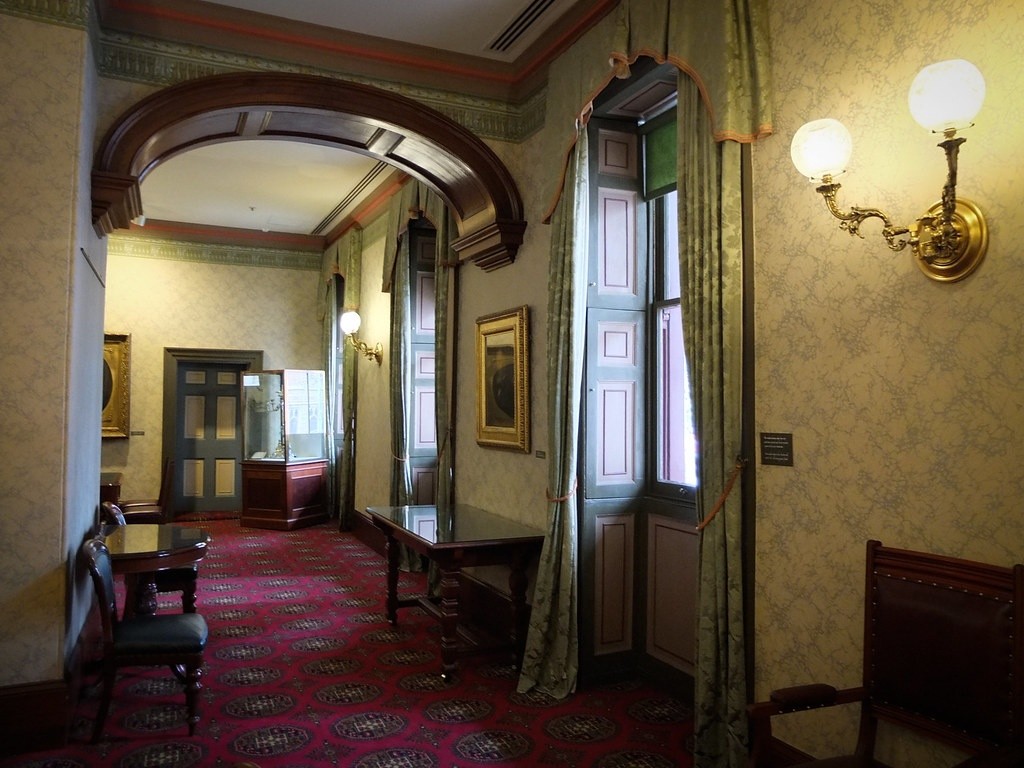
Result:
[102,331,133,440]
[475,305,531,454]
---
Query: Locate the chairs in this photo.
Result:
[102,501,199,617]
[744,531,1024,768]
[81,539,209,742]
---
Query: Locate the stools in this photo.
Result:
[121,506,162,525]
[122,499,158,508]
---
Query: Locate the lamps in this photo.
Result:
[790,59,990,283]
[340,311,383,365]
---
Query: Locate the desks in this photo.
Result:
[97,524,210,619]
[99,472,123,520]
[365,501,545,685]
